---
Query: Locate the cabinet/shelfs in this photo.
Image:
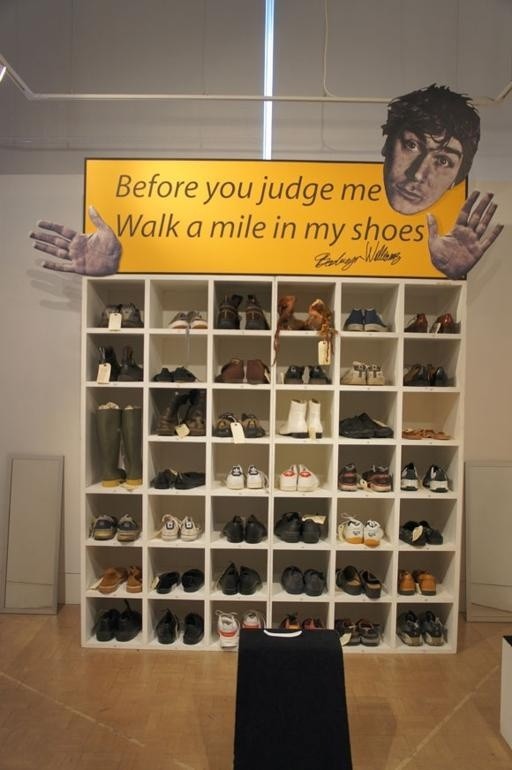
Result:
[80,272,469,655]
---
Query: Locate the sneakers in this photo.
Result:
[183,610,204,646]
[277,294,333,332]
[404,313,456,334]
[340,359,386,386]
[238,565,262,596]
[400,461,419,492]
[99,564,128,594]
[281,565,327,597]
[217,293,270,331]
[156,567,205,594]
[154,468,206,490]
[404,363,448,386]
[98,302,144,328]
[279,613,326,631]
[154,367,196,383]
[217,609,263,649]
[157,391,207,436]
[280,463,320,492]
[338,461,393,493]
[126,565,143,594]
[274,510,322,544]
[283,365,305,385]
[213,358,244,384]
[160,512,201,542]
[422,464,450,493]
[398,567,437,596]
[214,411,266,438]
[335,565,382,598]
[308,365,329,386]
[246,464,267,489]
[218,562,239,595]
[225,464,245,490]
[96,607,143,642]
[155,607,181,645]
[244,514,268,544]
[167,310,208,330]
[335,617,380,646]
[246,359,271,385]
[339,412,394,439]
[221,515,244,543]
[93,513,118,540]
[116,513,141,542]
[396,608,446,647]
[399,519,445,547]
[339,511,385,547]
[343,307,390,332]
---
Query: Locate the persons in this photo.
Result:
[381,83,480,215]
[29,206,121,276]
[425,190,504,279]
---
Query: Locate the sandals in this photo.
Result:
[402,427,452,440]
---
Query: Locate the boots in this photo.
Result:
[122,404,144,485]
[98,345,144,382]
[95,407,128,487]
[278,398,324,439]
